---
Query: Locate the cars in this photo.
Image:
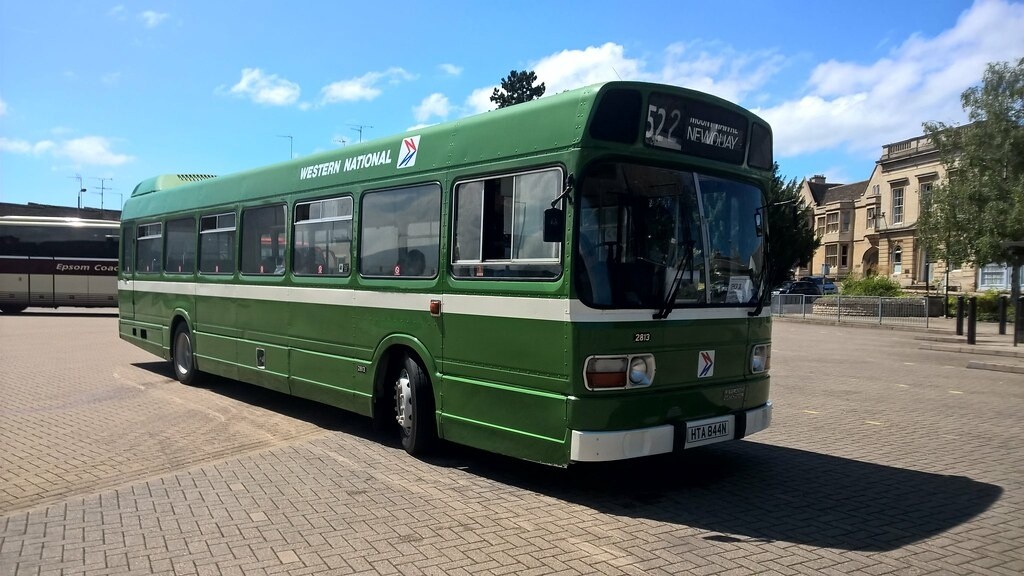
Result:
[773,281,820,304]
[801,275,838,295]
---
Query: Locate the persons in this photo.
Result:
[522,194,609,307]
[401,249,426,277]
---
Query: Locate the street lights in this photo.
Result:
[77,188,87,218]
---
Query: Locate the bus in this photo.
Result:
[0,216,123,315]
[120,80,815,470]
